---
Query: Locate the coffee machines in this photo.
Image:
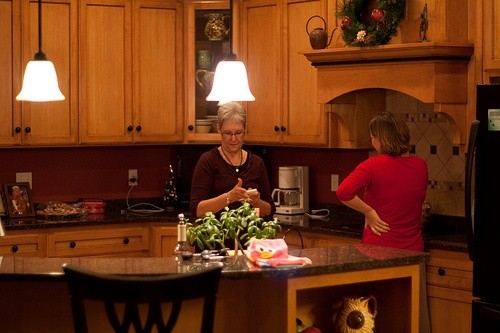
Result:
[274,165,309,213]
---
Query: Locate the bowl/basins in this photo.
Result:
[195,113,219,134]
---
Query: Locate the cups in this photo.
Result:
[197,49,215,66]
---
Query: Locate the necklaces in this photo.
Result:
[221,146,243,173]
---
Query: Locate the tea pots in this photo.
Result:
[306,14,337,49]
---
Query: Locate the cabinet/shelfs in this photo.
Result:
[0,219,474,333]
[0,0,500,149]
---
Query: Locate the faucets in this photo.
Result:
[282,227,305,249]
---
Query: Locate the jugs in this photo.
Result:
[204,13,229,41]
[272,188,300,208]
[195,69,215,95]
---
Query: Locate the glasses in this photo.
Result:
[223,130,245,137]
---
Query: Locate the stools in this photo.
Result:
[63,263,222,333]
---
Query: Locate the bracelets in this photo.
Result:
[225,192,231,205]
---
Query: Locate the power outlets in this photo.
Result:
[331,174,339,191]
[128,169,138,186]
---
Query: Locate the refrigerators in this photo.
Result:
[464,83,500,333]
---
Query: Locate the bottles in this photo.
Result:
[173,214,192,269]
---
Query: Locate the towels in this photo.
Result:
[243,237,312,269]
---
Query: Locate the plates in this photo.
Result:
[226,249,248,258]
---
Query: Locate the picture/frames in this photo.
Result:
[4,182,36,218]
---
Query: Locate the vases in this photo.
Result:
[337,295,377,333]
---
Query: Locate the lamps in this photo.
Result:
[205,0,255,101]
[16,0,66,102]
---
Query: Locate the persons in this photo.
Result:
[336,110,429,333]
[189,102,277,254]
